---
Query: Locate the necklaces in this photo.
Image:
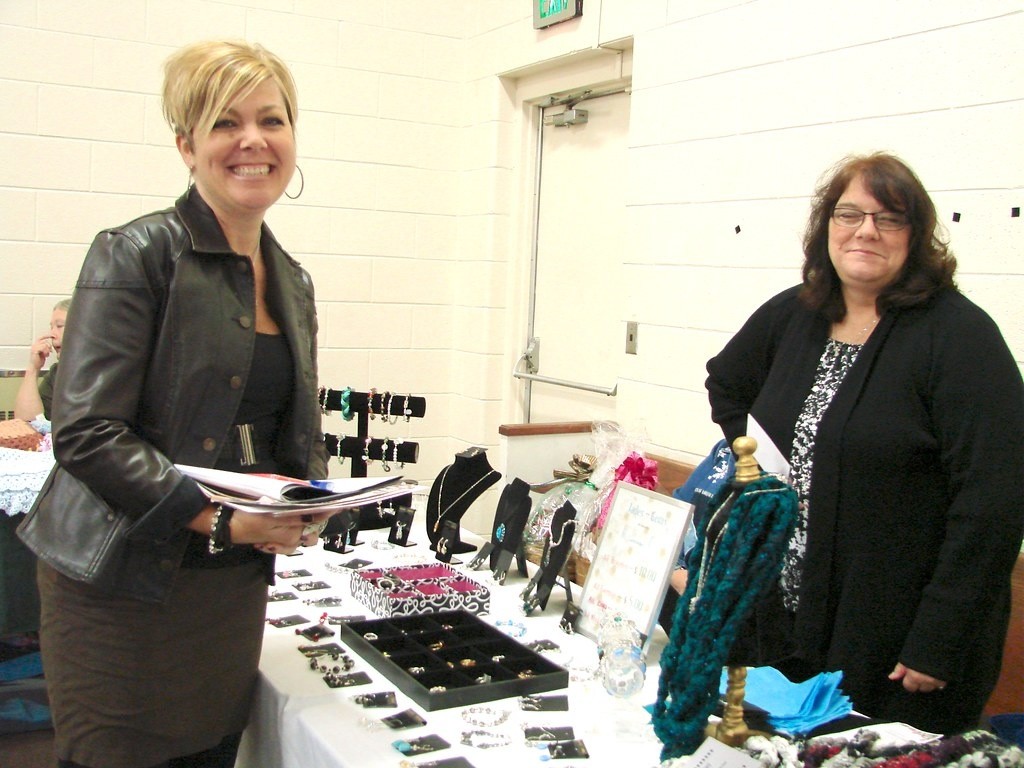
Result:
[435,463,495,533]
[688,491,739,616]
[544,519,577,565]
[833,316,877,343]
[496,523,506,542]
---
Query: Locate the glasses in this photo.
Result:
[830,206,910,231]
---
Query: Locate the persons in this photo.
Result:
[18,298,73,426]
[704,157,1024,733]
[15,37,331,768]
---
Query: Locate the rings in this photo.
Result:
[304,522,318,535]
[365,623,540,698]
[938,684,945,691]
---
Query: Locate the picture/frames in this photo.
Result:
[572,480,696,659]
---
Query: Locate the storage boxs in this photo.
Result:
[339,611,569,714]
[348,562,491,619]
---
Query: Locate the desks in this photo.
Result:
[218,530,945,768]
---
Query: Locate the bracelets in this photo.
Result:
[318,386,413,472]
[206,503,235,554]
[595,610,650,699]
